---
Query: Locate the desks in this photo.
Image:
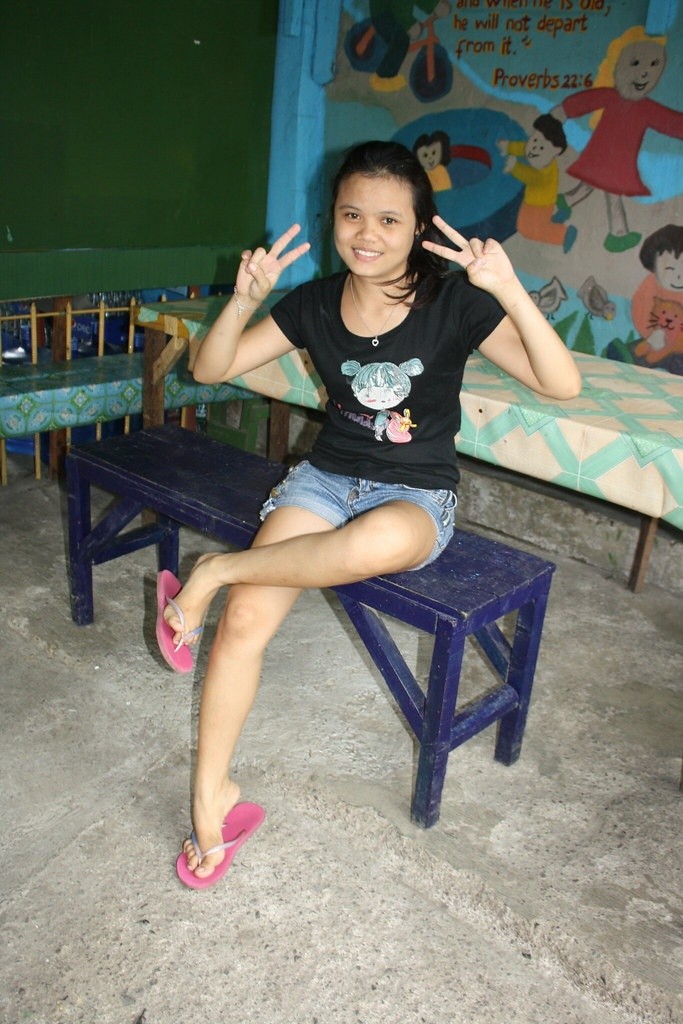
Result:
[132,287,683,593]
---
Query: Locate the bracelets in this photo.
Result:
[234,287,257,318]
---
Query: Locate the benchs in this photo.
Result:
[0,350,269,488]
[64,422,556,827]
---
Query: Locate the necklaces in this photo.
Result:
[350,278,406,346]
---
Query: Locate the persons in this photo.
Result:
[156,141,581,888]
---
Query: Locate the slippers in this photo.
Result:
[156,569,204,673]
[176,801,265,889]
